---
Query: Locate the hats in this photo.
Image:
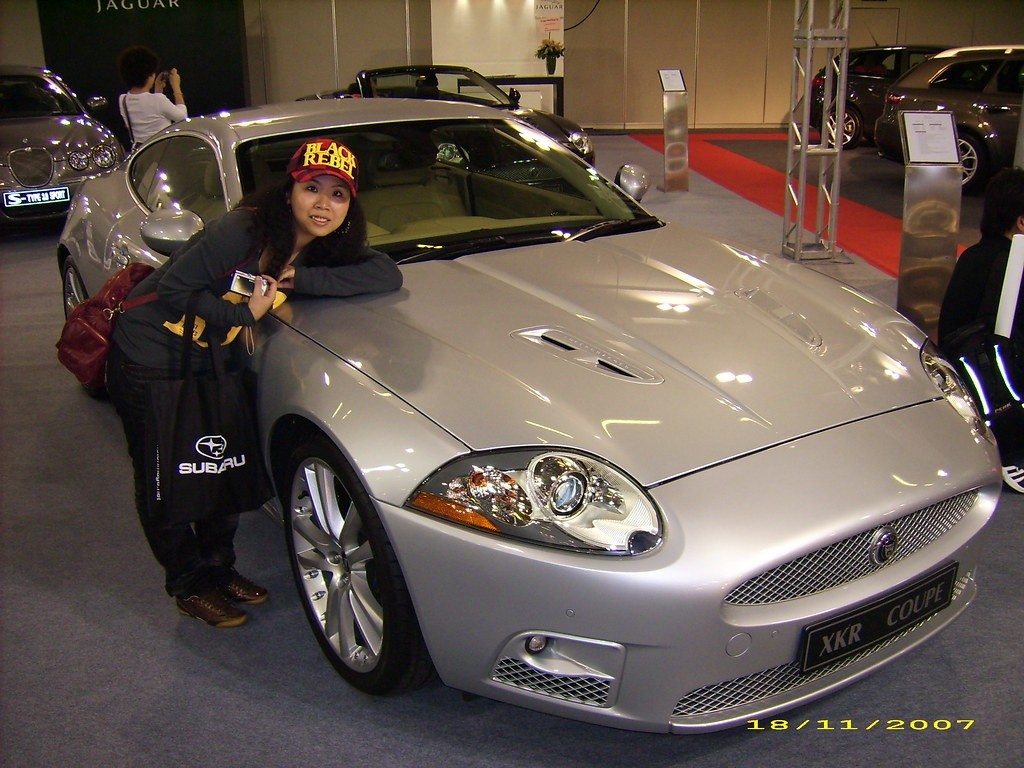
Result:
[287,137,359,198]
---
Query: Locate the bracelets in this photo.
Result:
[173,92,183,96]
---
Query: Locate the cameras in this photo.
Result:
[229,270,269,297]
[161,71,171,81]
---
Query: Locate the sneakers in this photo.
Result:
[175,590,248,628]
[210,566,269,604]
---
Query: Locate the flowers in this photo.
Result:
[533,39,565,60]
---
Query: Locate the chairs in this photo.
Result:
[185,157,228,224]
[358,155,464,230]
[348,82,360,98]
[415,76,439,101]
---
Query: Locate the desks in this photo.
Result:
[456,75,564,118]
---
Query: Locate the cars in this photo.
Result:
[0,63,127,225]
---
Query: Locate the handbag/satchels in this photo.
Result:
[54,262,156,383]
[146,297,276,530]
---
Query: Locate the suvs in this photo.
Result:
[872,43,1024,197]
[808,42,991,151]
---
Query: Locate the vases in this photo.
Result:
[546,56,557,75]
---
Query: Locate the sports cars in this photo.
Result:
[58,95,1007,737]
[295,63,597,197]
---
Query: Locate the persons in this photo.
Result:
[105,139,402,627]
[119,45,187,156]
[939,169,1024,493]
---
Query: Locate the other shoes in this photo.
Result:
[1001,463,1024,494]
